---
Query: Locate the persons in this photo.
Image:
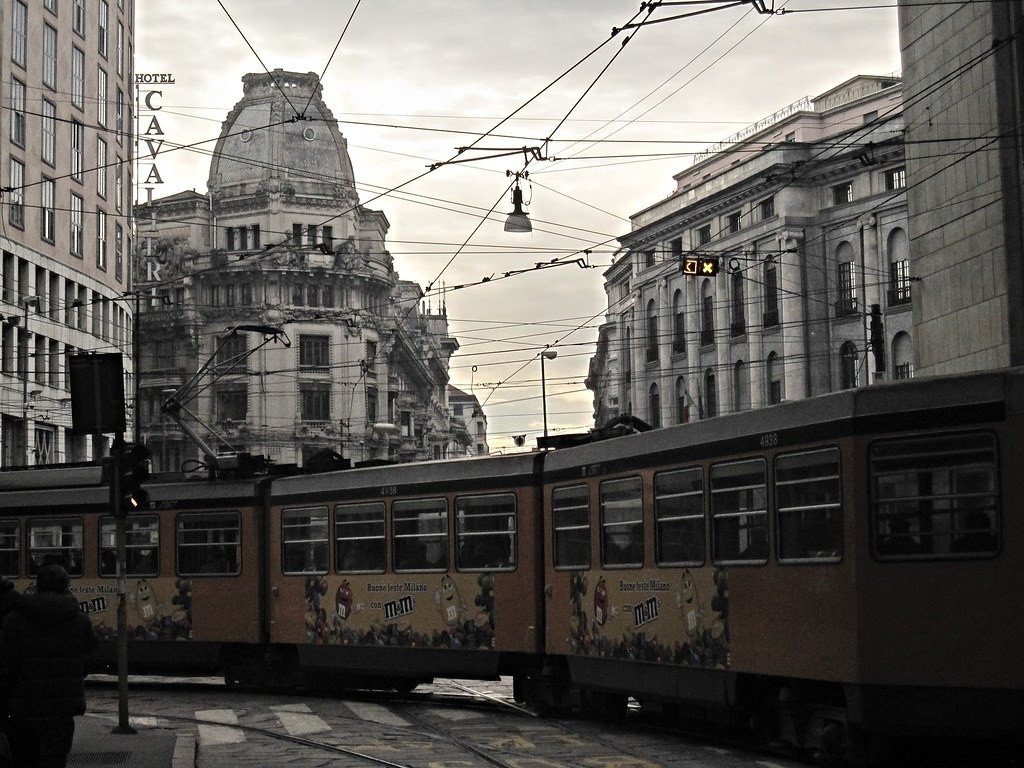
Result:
[1,563,95,768]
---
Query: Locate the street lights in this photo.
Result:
[541,351,558,450]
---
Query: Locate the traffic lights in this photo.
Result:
[682,258,719,276]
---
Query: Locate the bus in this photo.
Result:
[0,325,1024,768]
